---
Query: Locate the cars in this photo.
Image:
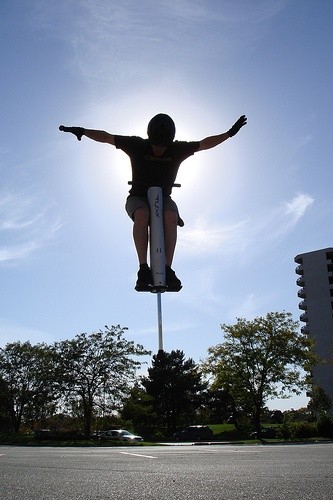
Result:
[91,430,144,446]
[250,429,282,440]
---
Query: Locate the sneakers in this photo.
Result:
[135,270,154,292]
[166,267,182,290]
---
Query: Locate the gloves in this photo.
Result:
[228,115,247,136]
[58,125,84,141]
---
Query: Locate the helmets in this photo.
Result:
[147,113,176,147]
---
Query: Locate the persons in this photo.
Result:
[57,112,247,299]
